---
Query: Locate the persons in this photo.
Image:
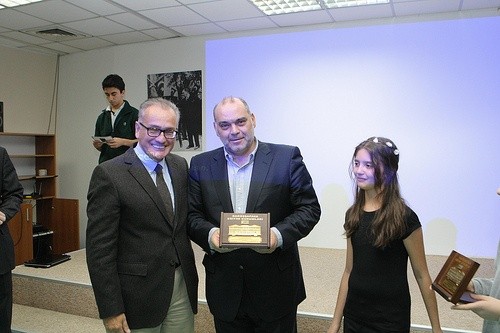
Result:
[0,146,24,333]
[86,98,199,333]
[327,137,443,333]
[181,88,200,150]
[93,74,139,164]
[449,188,500,333]
[188,96,321,333]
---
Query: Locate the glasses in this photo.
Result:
[138,121,179,139]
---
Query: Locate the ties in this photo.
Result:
[154,164,174,223]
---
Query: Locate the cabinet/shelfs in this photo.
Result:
[0,133,80,266]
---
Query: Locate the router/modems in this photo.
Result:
[31,181,43,199]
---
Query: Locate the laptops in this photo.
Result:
[24,254,71,268]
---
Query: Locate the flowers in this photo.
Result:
[373,137,400,155]
